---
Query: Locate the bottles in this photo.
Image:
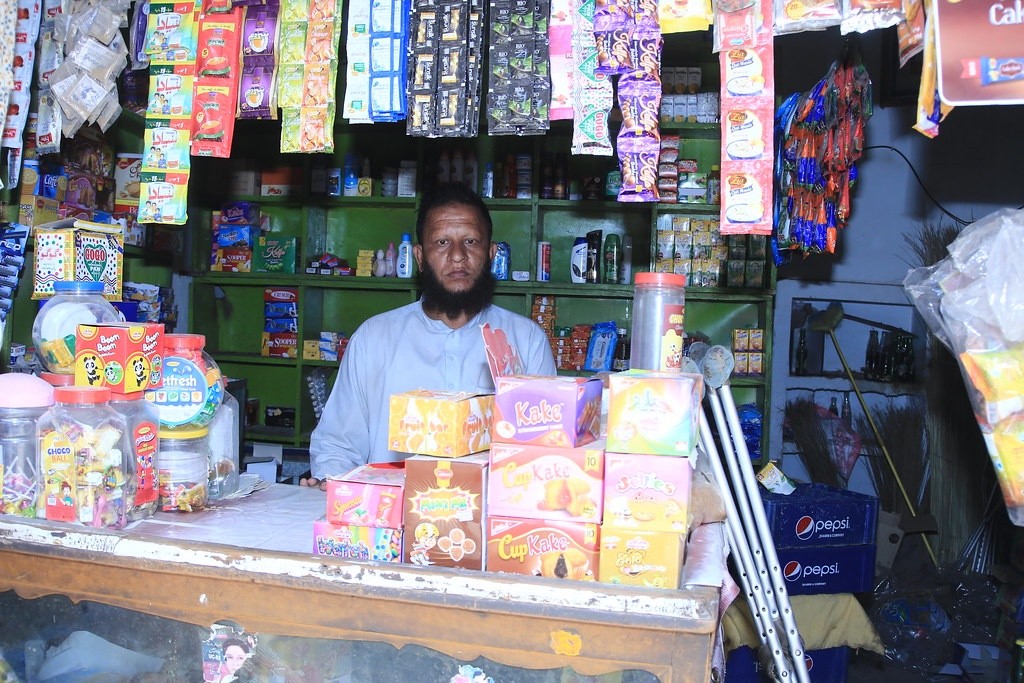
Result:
[797,327,808,376]
[373,250,386,277]
[540,152,554,199]
[614,328,630,372]
[829,396,838,419]
[386,241,396,277]
[554,165,568,199]
[495,163,503,197]
[503,154,516,197]
[683,337,705,362]
[123,56,140,101]
[344,155,358,195]
[396,232,413,278]
[841,392,852,428]
[867,330,915,382]
[482,162,494,198]
[708,165,720,205]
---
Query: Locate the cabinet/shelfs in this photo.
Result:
[0,91,184,374]
[770,278,931,496]
[180,118,778,470]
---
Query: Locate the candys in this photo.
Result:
[0,455,207,530]
[38,334,76,375]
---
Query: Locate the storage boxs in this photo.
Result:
[18,108,372,483]
[777,543,877,596]
[653,66,767,376]
[758,482,880,549]
[313,513,404,563]
[388,386,494,459]
[605,366,702,458]
[486,516,600,581]
[952,643,999,676]
[603,454,694,535]
[487,441,604,522]
[876,513,939,571]
[396,160,432,198]
[325,462,406,529]
[600,526,687,590]
[492,373,603,449]
[724,646,848,683]
[401,451,489,571]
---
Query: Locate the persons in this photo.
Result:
[222,638,251,674]
[300,181,559,491]
